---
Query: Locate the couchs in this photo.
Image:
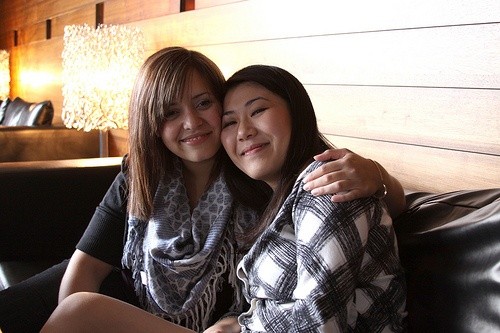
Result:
[0,96,55,127]
[0,156,499,333]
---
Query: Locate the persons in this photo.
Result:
[37,60,408,333]
[0,46,407,333]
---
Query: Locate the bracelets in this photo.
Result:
[369,159,387,199]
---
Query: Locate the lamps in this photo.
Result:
[62,23,146,158]
[0,49,11,100]
